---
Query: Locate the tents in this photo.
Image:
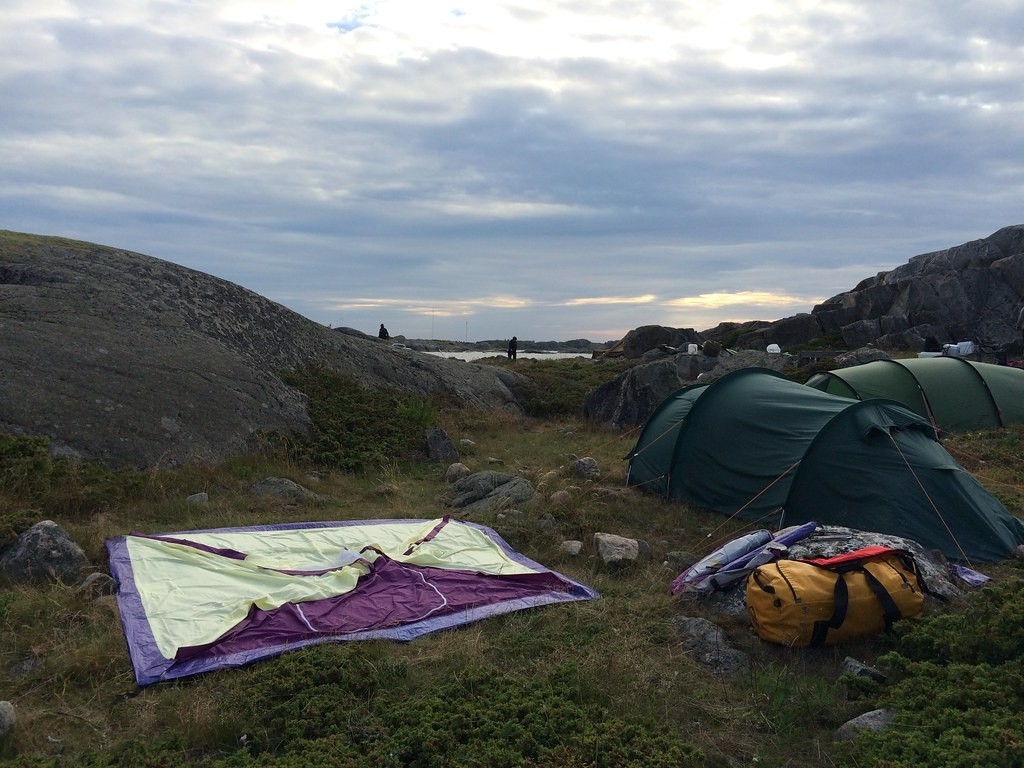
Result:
[560,366,1024,564]
[804,356,1024,431]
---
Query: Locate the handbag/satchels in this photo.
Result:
[746,546,930,650]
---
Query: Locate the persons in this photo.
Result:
[508,337,517,361]
[379,324,388,340]
